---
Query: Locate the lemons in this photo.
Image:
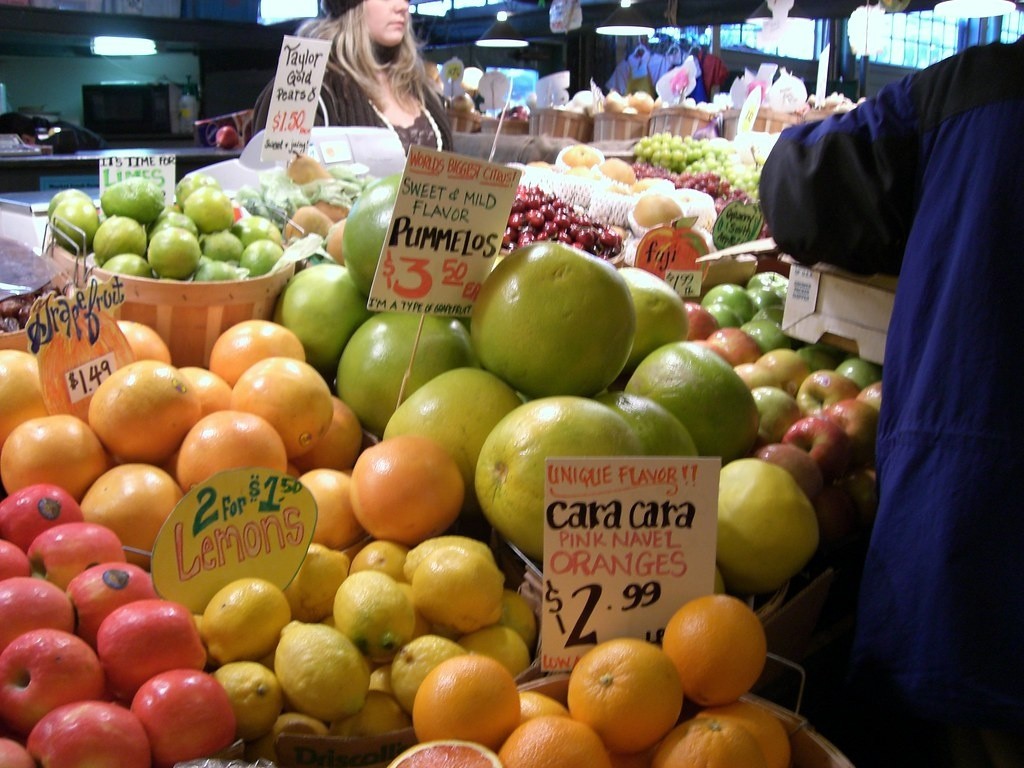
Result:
[46,170,285,282]
[190,535,533,759]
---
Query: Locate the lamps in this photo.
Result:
[594,3,656,36]
[474,10,530,48]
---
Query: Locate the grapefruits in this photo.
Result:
[276,174,821,598]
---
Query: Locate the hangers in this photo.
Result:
[687,37,704,58]
[664,38,681,56]
[631,36,648,56]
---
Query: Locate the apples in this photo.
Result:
[666,269,886,547]
[0,483,235,768]
[521,143,682,236]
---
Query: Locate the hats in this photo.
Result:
[322,0,365,20]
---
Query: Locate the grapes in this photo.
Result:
[622,132,777,239]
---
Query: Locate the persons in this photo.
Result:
[244,1,452,153]
[0,113,112,155]
[761,28,1024,768]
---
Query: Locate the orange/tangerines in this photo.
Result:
[0,316,463,567]
[385,594,797,768]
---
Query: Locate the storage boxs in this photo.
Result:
[780,263,898,364]
[447,109,482,134]
[528,110,592,143]
[804,110,849,120]
[592,114,648,143]
[481,120,529,135]
[50,221,296,371]
[723,107,802,141]
[648,107,718,138]
[0,266,71,354]
